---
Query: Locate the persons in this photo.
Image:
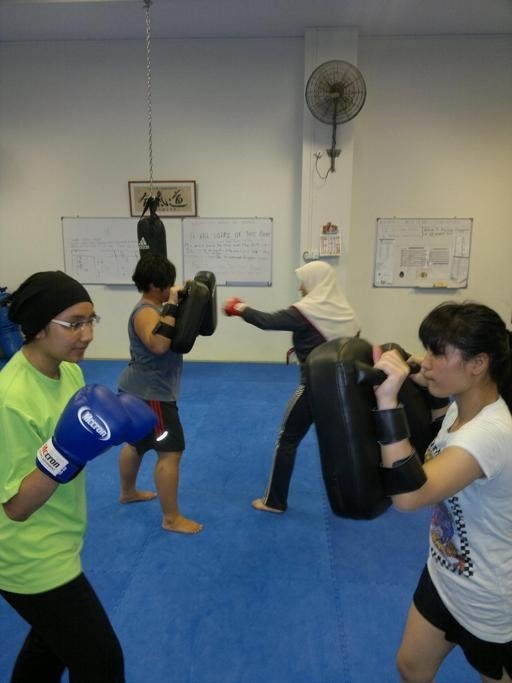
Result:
[225,262,360,515]
[371,300,512,683]
[0,270,156,681]
[111,255,203,536]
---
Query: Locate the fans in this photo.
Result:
[303,56,367,171]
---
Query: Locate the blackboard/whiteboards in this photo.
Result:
[62,216,142,287]
[182,217,275,286]
[372,215,474,290]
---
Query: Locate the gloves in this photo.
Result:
[113,388,158,445]
[34,381,133,484]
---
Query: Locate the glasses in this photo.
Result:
[51,314,100,327]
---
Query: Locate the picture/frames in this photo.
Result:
[127,179,198,218]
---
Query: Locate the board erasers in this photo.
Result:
[432,281,448,288]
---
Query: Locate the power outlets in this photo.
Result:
[315,149,324,158]
